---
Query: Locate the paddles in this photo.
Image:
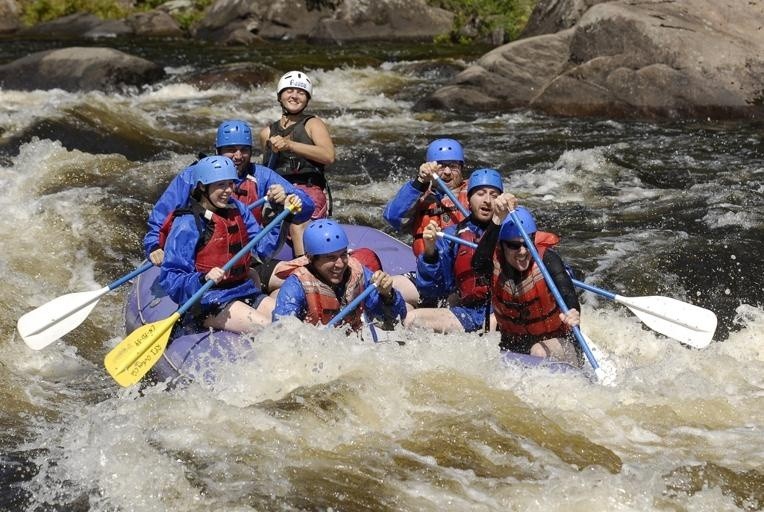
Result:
[18,195,270,352]
[435,230,718,349]
[103,203,295,388]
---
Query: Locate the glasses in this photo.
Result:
[503,240,534,249]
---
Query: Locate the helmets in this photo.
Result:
[303,218,349,258]
[497,207,537,240]
[192,155,241,190]
[277,70,313,98]
[425,138,465,166]
[216,119,252,147]
[467,169,503,196]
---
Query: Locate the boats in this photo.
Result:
[123,223,593,395]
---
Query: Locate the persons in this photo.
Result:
[469,192,587,369]
[389,168,576,344]
[382,139,475,315]
[260,68,334,223]
[159,156,303,339]
[268,216,406,351]
[141,119,316,297]
